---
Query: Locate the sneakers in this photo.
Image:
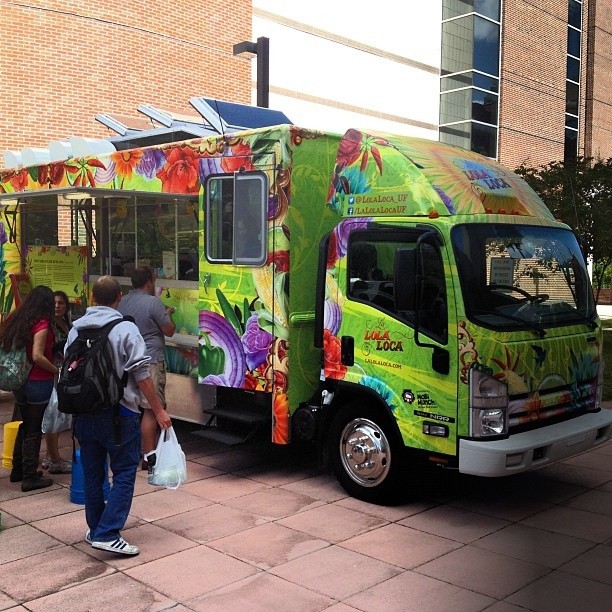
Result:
[48,459,73,474]
[40,457,50,470]
[88,538,140,557]
[84,529,92,544]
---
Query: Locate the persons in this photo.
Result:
[115,266,175,470]
[40,290,72,474]
[63,275,171,555]
[0,285,57,492]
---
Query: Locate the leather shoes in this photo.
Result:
[20,479,53,492]
[142,460,149,470]
[10,470,43,482]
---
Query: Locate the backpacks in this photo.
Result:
[0,332,32,391]
[56,315,137,465]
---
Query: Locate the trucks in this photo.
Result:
[0,96,612,509]
